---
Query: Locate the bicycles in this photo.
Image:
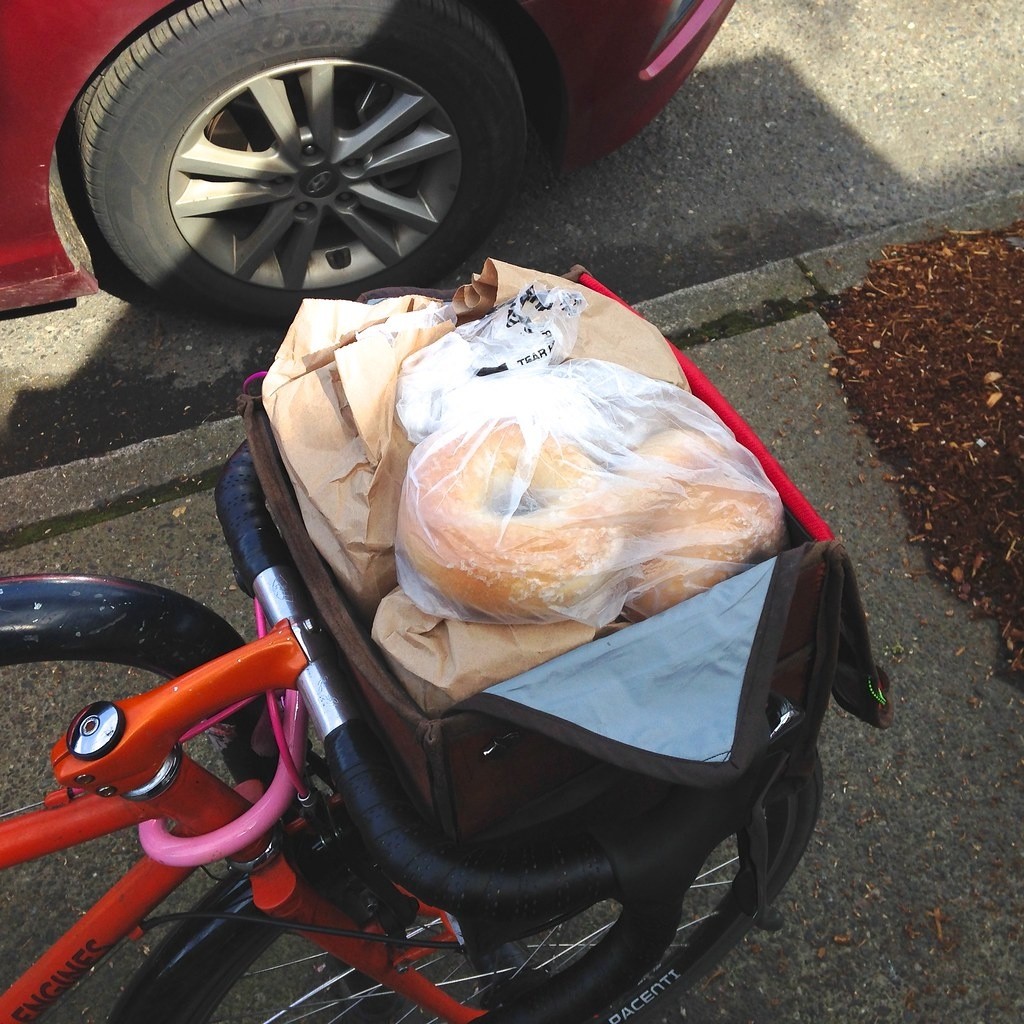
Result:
[6,258,888,1019]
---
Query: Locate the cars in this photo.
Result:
[3,0,740,320]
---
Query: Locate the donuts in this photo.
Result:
[399,417,787,624]
